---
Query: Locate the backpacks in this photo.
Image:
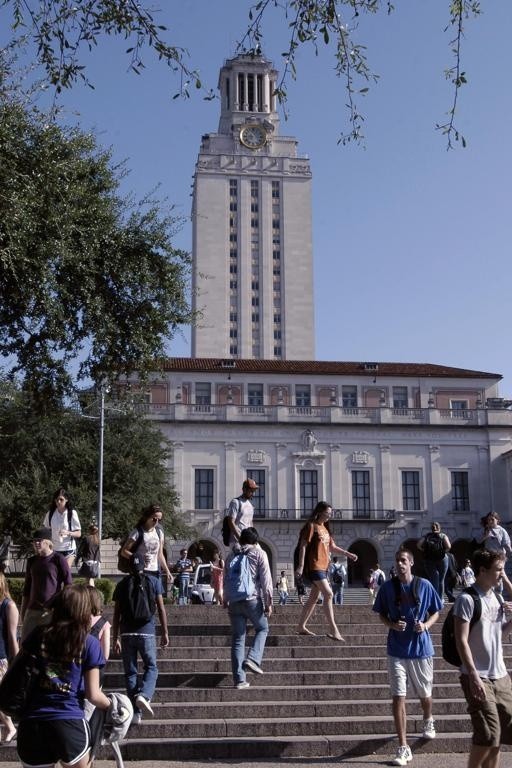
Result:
[221,497,241,546]
[441,587,503,668]
[332,564,344,583]
[117,526,161,573]
[375,569,385,585]
[0,623,49,716]
[118,572,157,628]
[294,520,332,576]
[422,532,446,564]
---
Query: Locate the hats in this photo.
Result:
[242,478,259,491]
[27,529,52,542]
[128,551,145,571]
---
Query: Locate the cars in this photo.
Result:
[192,563,219,605]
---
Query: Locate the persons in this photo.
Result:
[417,522,458,603]
[471,511,512,595]
[277,571,290,605]
[294,556,347,605]
[222,479,273,689]
[295,501,357,642]
[457,558,475,589]
[367,563,397,604]
[453,549,512,768]
[174,548,224,606]
[372,549,444,765]
[1,489,173,768]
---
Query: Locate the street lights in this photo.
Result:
[83,381,123,572]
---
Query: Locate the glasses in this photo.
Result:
[151,515,162,524]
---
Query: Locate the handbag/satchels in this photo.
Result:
[453,573,463,585]
[173,576,180,587]
[77,563,99,578]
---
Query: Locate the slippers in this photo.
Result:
[3,727,17,745]
[295,630,315,636]
[326,632,344,641]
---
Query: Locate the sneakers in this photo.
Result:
[136,695,153,717]
[133,715,141,724]
[392,744,413,766]
[234,681,249,690]
[422,714,435,740]
[244,660,263,675]
